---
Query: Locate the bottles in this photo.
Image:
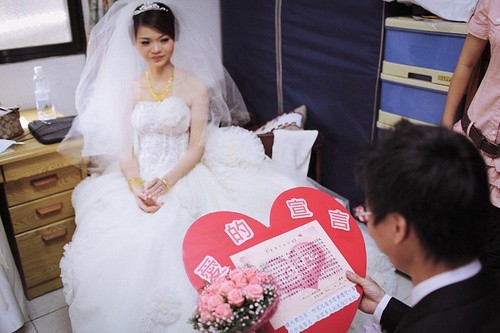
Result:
[34,66,56,120]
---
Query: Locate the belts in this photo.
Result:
[461,111,500,158]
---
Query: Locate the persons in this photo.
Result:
[344,125,500,333]
[441,0,500,221]
[59,0,265,333]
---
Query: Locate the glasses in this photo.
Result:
[354,202,372,221]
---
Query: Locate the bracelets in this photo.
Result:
[160,177,169,191]
[126,176,144,191]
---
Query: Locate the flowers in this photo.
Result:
[187,265,279,333]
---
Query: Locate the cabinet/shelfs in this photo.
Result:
[0,107,90,297]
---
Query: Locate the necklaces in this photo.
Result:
[145,64,177,102]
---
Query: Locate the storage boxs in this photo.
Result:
[377,14,473,152]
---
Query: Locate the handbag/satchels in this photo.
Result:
[0,105,24,139]
